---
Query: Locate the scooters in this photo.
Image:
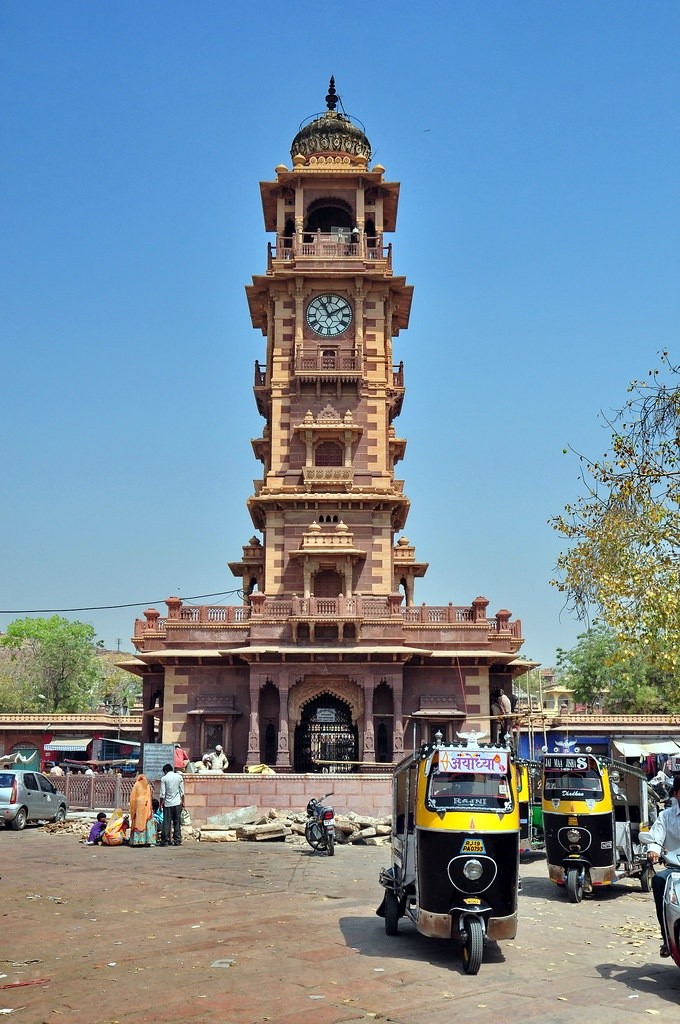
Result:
[636,831,680,969]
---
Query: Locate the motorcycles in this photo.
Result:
[540,737,653,903]
[305,792,337,856]
[376,729,530,976]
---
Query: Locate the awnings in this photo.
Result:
[612,738,680,759]
[44,737,93,752]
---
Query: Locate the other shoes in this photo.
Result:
[159,844,168,847]
[175,843,182,846]
[660,943,670,958]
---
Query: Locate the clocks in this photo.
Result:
[306,293,353,337]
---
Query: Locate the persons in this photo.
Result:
[194,744,229,773]
[48,761,123,778]
[491,687,511,717]
[129,774,158,847]
[173,743,189,773]
[645,773,680,958]
[88,813,107,846]
[103,808,130,846]
[160,764,187,847]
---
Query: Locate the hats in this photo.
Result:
[215,745,223,751]
[202,754,212,762]
[175,743,181,747]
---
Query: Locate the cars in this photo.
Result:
[0,769,70,831]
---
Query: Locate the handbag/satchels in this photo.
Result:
[180,808,190,825]
